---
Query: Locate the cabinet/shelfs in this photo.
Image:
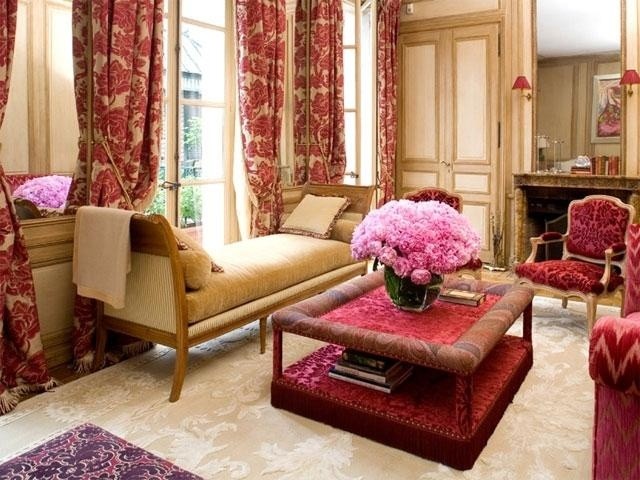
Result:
[15,215,76,368]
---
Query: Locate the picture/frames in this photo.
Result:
[591,73,621,143]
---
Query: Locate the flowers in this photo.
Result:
[14,175,73,217]
[351,199,482,284]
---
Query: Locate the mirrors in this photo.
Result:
[532,0,627,172]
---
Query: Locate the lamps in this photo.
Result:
[537,137,550,160]
[619,70,640,97]
[512,75,531,100]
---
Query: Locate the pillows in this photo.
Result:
[178,250,212,289]
[170,225,224,275]
[278,193,350,239]
[284,213,364,224]
[277,213,358,243]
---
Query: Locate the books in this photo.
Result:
[328,349,415,394]
[438,286,487,307]
[570,157,619,174]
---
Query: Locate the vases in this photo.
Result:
[384,269,442,312]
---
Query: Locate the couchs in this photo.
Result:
[512,195,636,340]
[68,181,379,403]
[589,223,640,478]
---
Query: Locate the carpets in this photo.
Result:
[0,421,204,480]
[1,295,622,478]
